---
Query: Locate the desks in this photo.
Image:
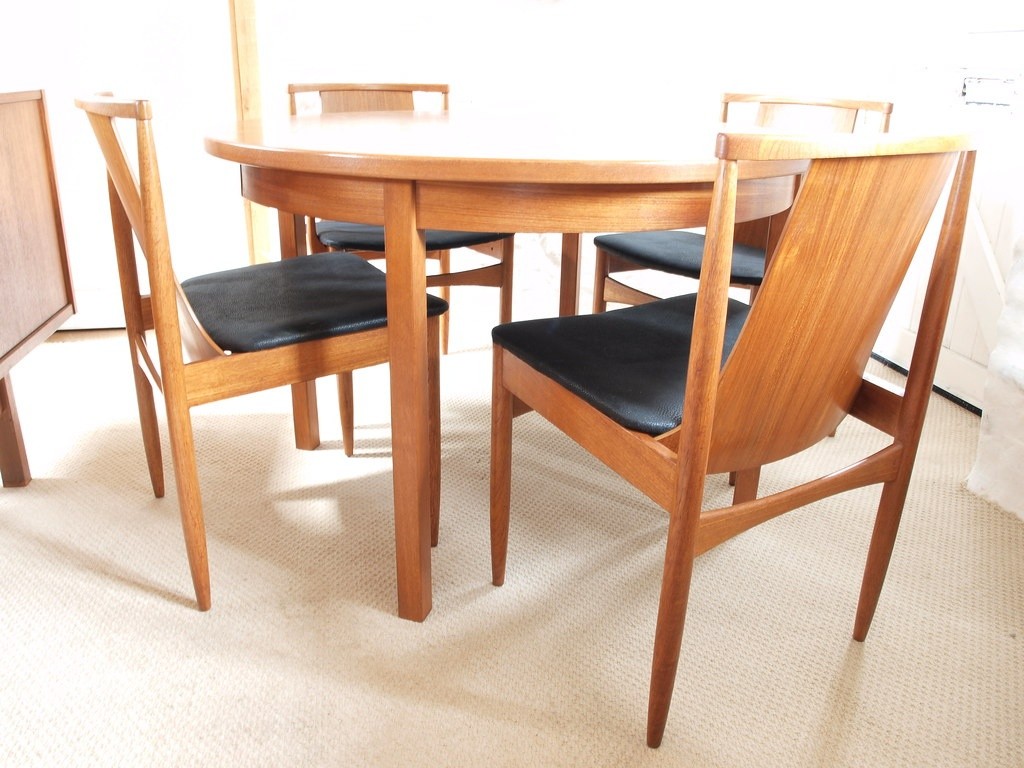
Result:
[204,107,821,621]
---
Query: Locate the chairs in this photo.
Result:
[294,82,516,456]
[488,123,980,751]
[595,91,898,485]
[72,90,444,609]
